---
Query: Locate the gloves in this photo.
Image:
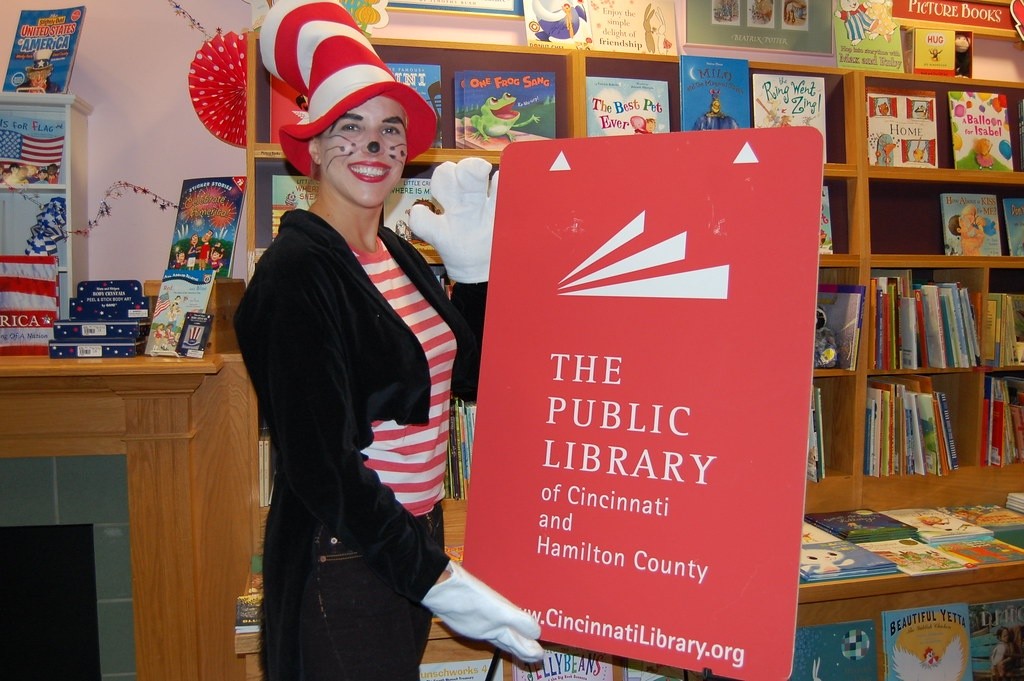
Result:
[404,156,501,287]
[420,559,548,671]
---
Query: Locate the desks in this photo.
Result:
[0,351,262,681]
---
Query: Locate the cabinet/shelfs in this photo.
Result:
[230,2,1024,679]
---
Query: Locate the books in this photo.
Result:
[1,3,85,93]
[145,267,218,357]
[270,174,449,263]
[168,174,248,278]
[447,393,479,501]
[797,270,1024,680]
[267,60,556,147]
[581,0,1024,256]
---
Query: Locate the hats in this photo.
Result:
[260,0,439,178]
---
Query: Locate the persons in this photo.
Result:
[232,2,543,680]
